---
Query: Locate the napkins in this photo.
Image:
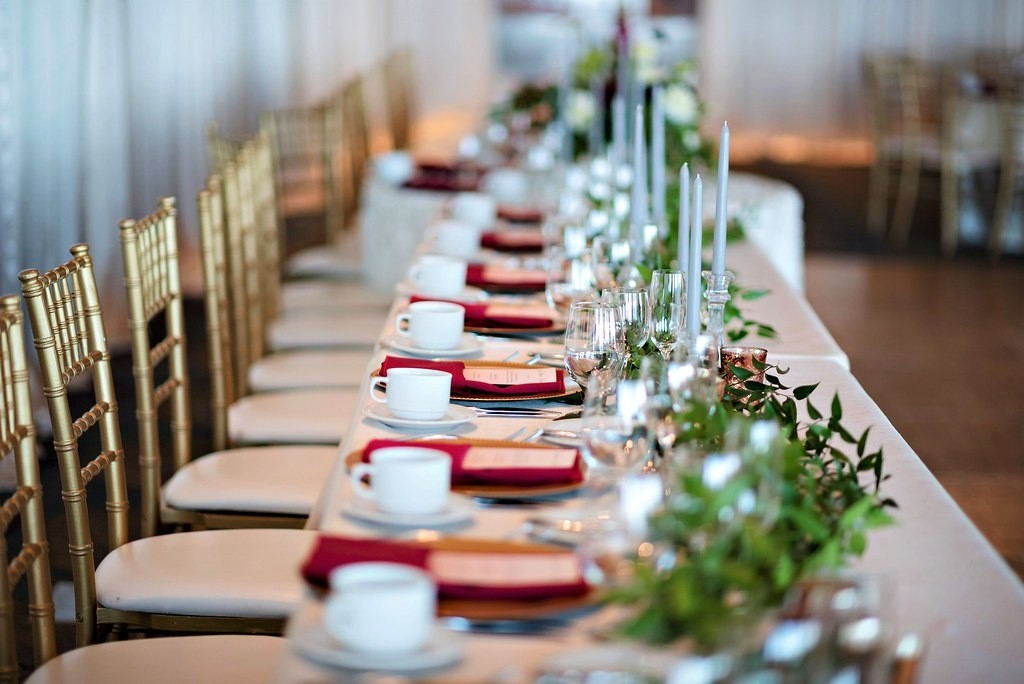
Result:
[479,226,545,253]
[302,531,591,603]
[408,297,548,327]
[380,354,569,398]
[465,261,563,296]
[493,204,545,222]
[362,438,583,488]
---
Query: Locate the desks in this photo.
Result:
[281,169,1024,684]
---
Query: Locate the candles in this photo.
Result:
[687,177,705,334]
[651,85,667,234]
[677,162,694,274]
[712,123,730,274]
[631,105,649,229]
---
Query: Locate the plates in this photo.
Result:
[447,286,489,301]
[387,334,480,357]
[363,401,478,430]
[342,493,473,528]
[292,632,458,671]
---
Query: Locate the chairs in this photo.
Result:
[228,148,386,346]
[127,194,339,541]
[196,177,361,447]
[22,243,306,644]
[860,43,1024,259]
[270,104,405,282]
[0,297,283,684]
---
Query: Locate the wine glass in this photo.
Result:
[547,223,712,594]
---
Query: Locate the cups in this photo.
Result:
[351,447,454,514]
[394,300,466,351]
[438,224,476,260]
[368,368,452,420]
[408,256,467,296]
[323,562,436,651]
